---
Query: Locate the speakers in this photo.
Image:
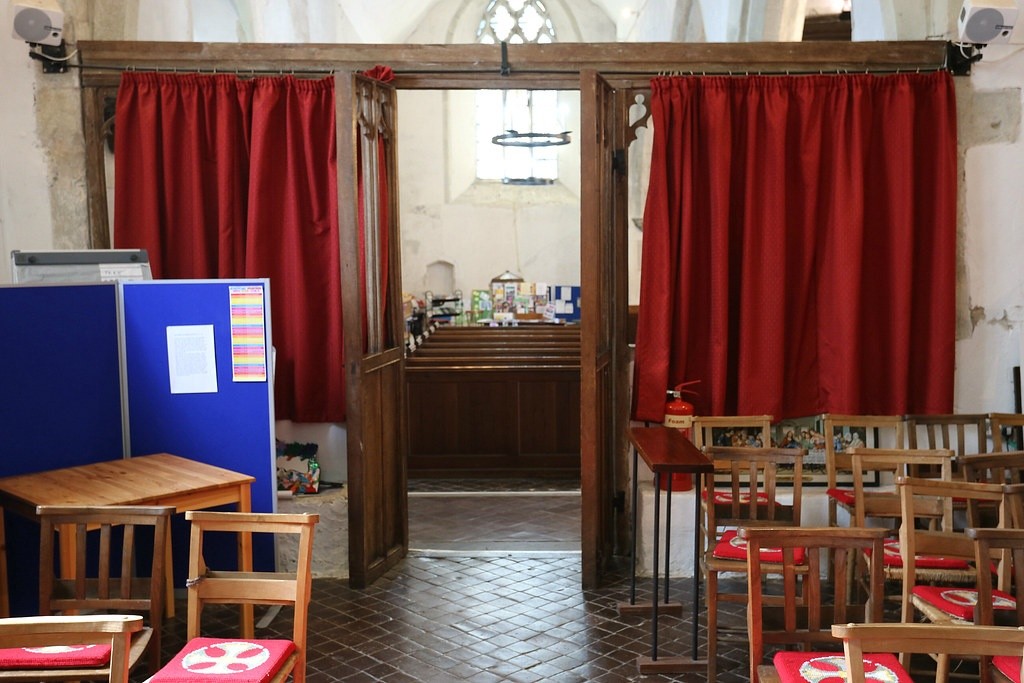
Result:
[12,4,64,46]
[958,0,1017,45]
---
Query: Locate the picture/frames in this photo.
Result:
[702,414,878,488]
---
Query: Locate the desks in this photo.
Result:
[0,454,256,642]
[618,427,714,674]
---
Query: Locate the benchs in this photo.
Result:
[403,320,582,480]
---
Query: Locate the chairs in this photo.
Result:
[0,505,321,683]
[690,412,1024,683]
